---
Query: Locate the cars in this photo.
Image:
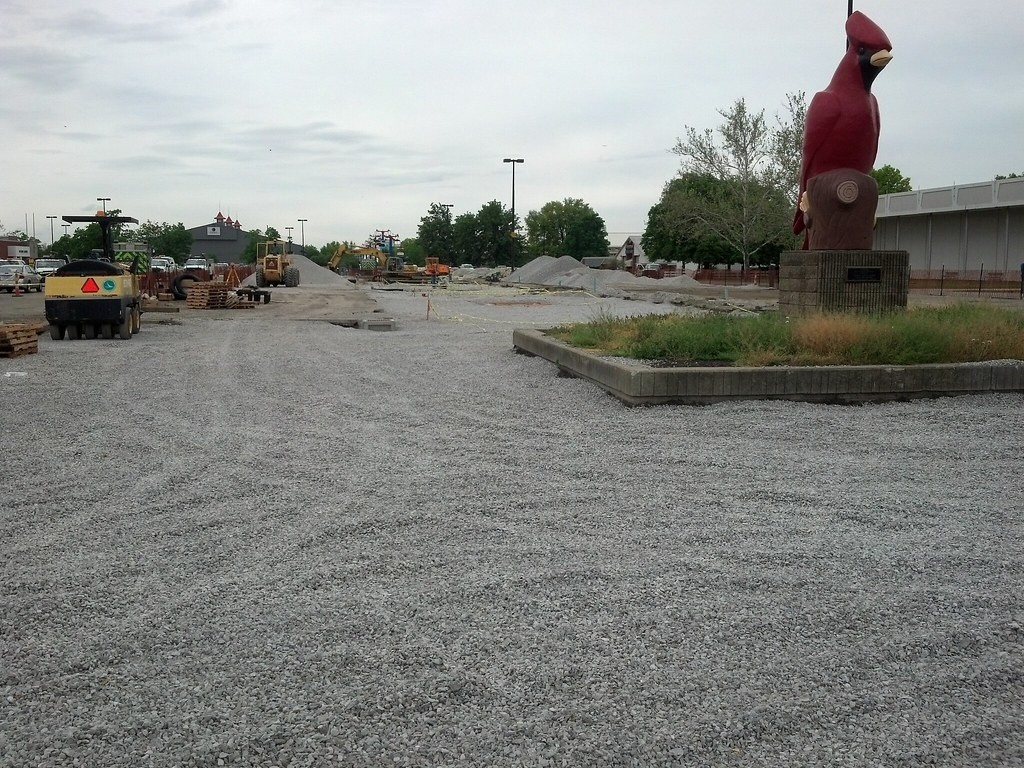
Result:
[150,256,176,274]
[0,259,67,292]
[459,264,473,269]
[184,259,209,271]
[637,263,664,278]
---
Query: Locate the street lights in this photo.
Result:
[285,227,294,254]
[298,220,308,256]
[61,225,70,235]
[97,198,111,216]
[441,204,454,225]
[503,158,524,273]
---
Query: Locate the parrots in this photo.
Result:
[793,11,893,251]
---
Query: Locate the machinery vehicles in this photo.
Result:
[45,260,143,340]
[326,241,419,279]
[419,257,450,276]
[256,239,299,288]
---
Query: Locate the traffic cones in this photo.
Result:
[46,216,57,243]
